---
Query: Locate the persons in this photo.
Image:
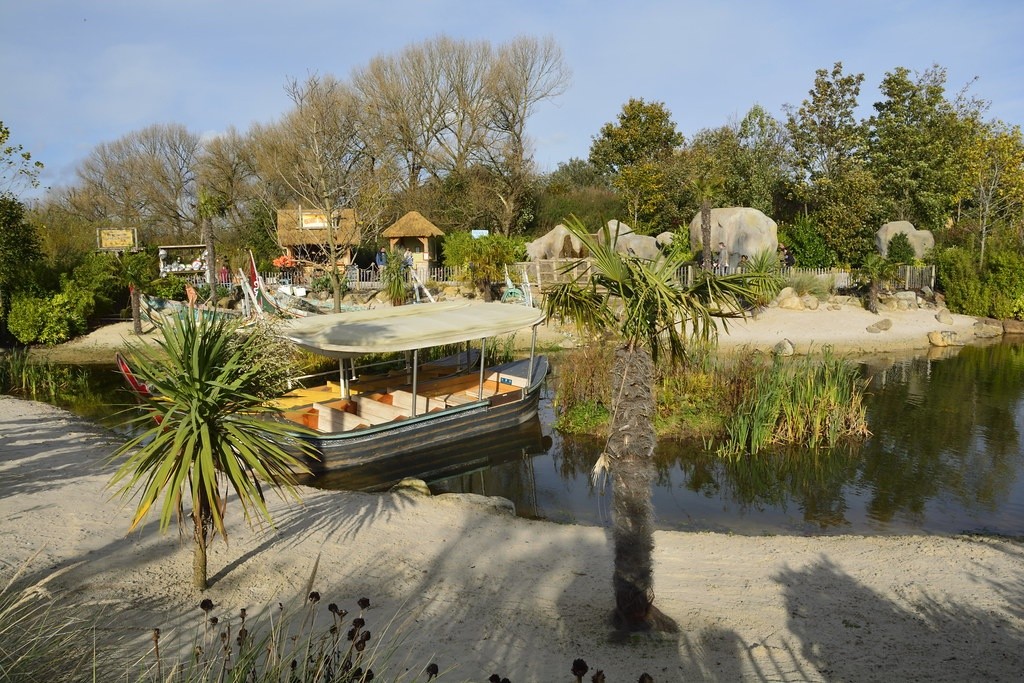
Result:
[777,242,795,273]
[400,248,414,283]
[693,247,714,270]
[735,255,753,274]
[715,241,729,277]
[219,255,232,288]
[375,246,388,282]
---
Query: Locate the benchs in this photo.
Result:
[387,387,447,416]
[313,403,374,433]
[326,380,373,397]
[469,387,505,400]
[389,370,439,383]
[358,375,408,389]
[350,394,419,426]
[434,393,473,407]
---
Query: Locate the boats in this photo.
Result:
[116,300,550,473]
[246,251,329,318]
[129,283,242,328]
[266,413,552,496]
